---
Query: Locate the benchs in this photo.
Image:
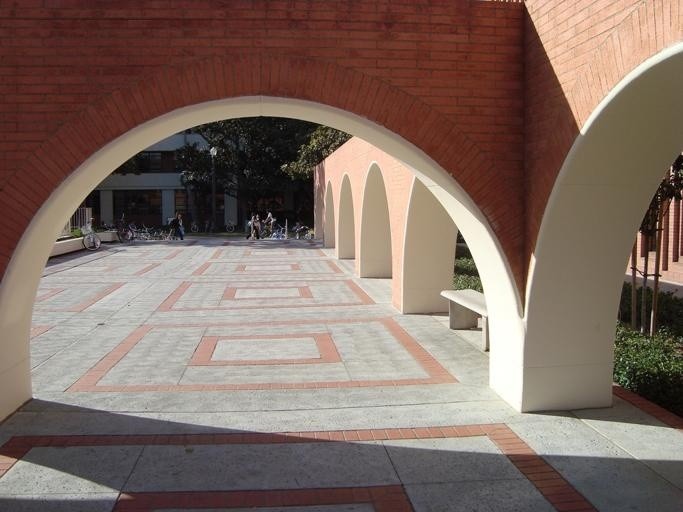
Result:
[441,288,490,353]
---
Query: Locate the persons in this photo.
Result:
[245,207,310,240]
[111,211,184,242]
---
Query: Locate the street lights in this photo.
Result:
[210,147,219,229]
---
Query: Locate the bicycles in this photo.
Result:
[226,218,235,232]
[83,218,101,250]
[114,213,135,242]
[191,221,199,232]
